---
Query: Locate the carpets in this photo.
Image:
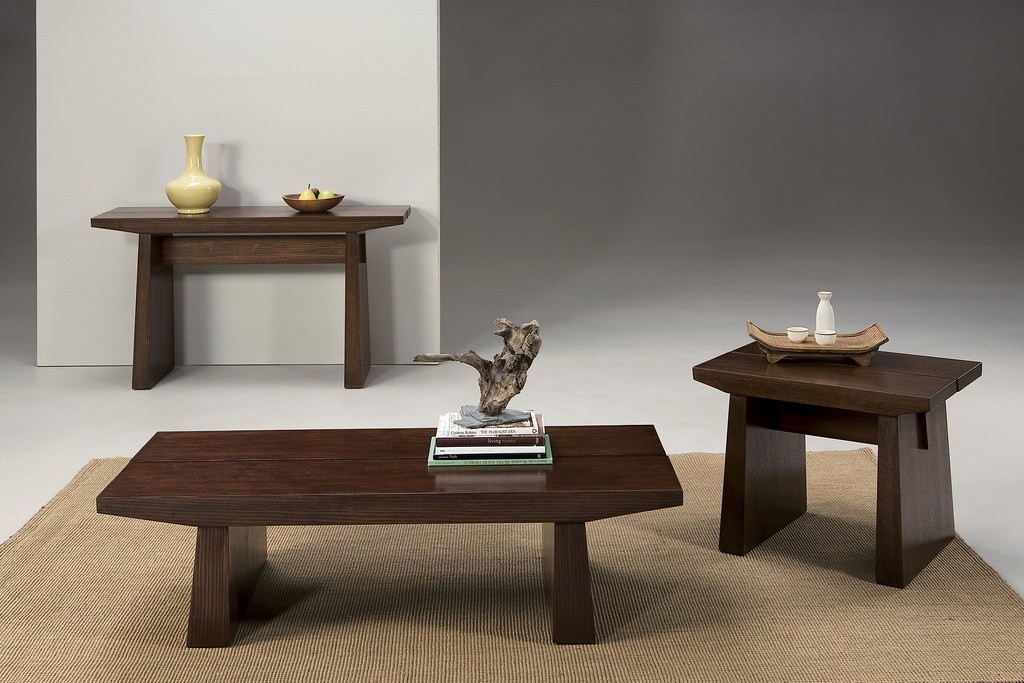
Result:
[0,446,1024,683]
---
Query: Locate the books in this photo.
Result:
[428,434,553,466]
[433,411,548,460]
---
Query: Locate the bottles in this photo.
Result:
[815,291,834,331]
[165,135,222,214]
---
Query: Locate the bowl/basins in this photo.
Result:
[282,193,345,213]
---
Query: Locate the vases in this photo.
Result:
[165,134,222,214]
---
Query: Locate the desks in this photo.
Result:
[91,205,410,390]
[692,341,983,588]
[96,425,684,649]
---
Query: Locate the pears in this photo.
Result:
[298,183,335,200]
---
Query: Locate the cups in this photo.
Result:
[787,327,809,342]
[814,330,837,345]
